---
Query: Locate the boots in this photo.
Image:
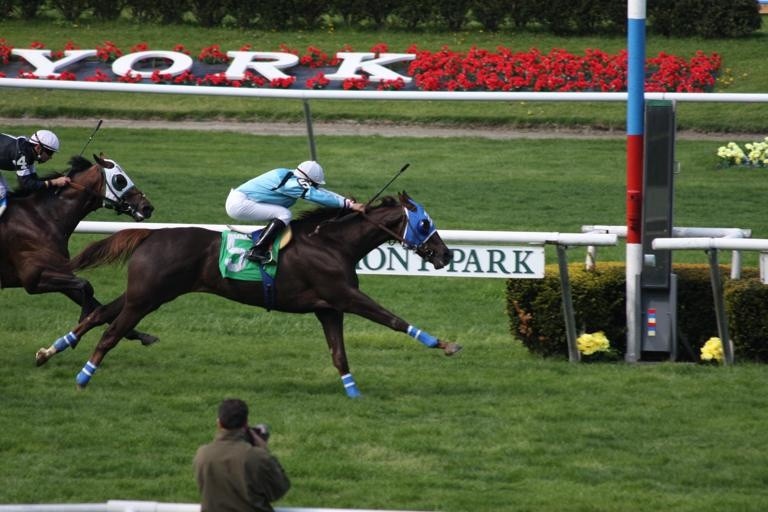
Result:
[246,218,287,266]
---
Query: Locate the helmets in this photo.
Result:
[29,129,60,153]
[294,161,327,185]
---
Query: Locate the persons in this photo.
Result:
[224,159,365,266]
[192,398,292,512]
[0,129,71,216]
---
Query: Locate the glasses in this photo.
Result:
[35,131,54,156]
[297,169,320,187]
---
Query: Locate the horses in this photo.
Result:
[35,189,463,401]
[0,151,159,346]
